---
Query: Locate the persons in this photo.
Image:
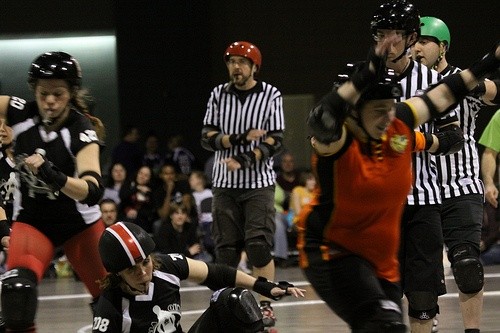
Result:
[368,0,500,333]
[90,220,306,333]
[99,122,321,266]
[296,31,500,333]
[0,52,110,333]
[200,41,286,325]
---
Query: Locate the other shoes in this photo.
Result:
[260,304,275,326]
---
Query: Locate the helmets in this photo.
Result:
[334,60,403,103]
[415,16,451,50]
[369,0,421,31]
[99,221,156,274]
[30,51,81,84]
[224,42,261,73]
